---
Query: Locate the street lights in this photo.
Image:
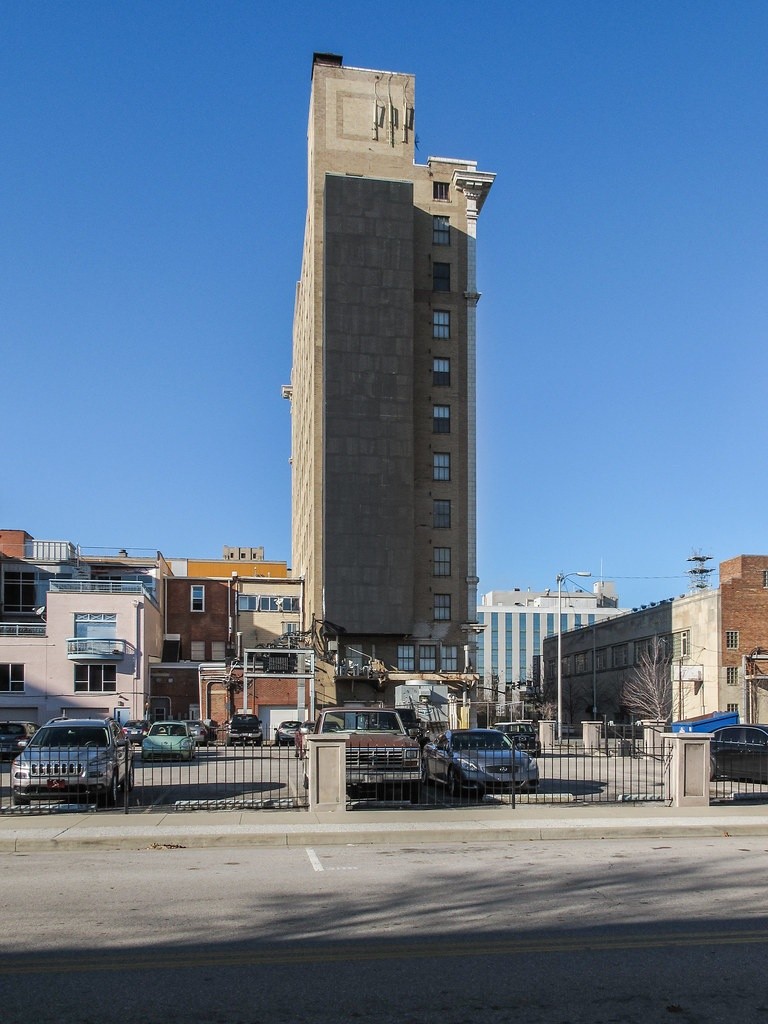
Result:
[558,572,591,744]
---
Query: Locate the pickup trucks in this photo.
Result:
[224,714,264,746]
[302,707,423,803]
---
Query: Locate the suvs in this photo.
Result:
[11,716,135,806]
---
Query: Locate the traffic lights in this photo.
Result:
[517,681,520,689]
[512,682,515,690]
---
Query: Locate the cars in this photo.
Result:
[123,720,152,746]
[294,720,342,759]
[709,724,768,782]
[171,720,210,746]
[0,720,41,760]
[490,722,541,758]
[365,708,421,737]
[421,728,541,798]
[142,721,196,761]
[274,721,303,746]
